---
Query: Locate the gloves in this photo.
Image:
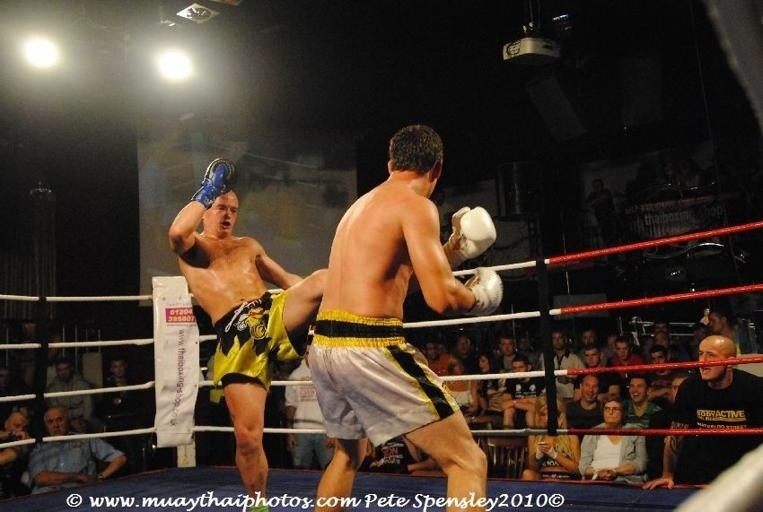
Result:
[462,266,507,318]
[440,205,499,268]
[189,157,239,211]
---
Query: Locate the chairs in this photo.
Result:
[480,436,526,479]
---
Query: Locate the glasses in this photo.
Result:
[604,406,622,414]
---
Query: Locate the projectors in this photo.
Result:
[500,38,561,66]
